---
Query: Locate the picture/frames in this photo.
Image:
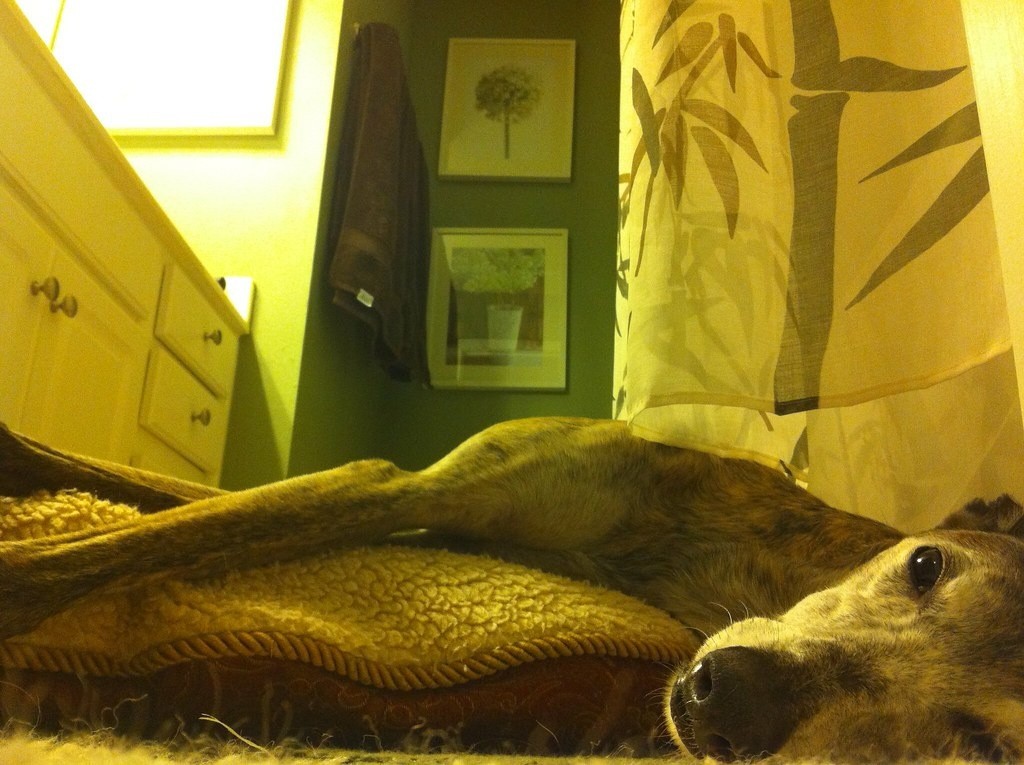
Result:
[436,36,577,184]
[50,0,298,140]
[426,226,569,392]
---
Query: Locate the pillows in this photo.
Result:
[1,484,702,751]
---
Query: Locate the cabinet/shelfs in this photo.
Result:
[0,0,252,489]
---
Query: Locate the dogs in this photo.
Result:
[0,416,1024,764]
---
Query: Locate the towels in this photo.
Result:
[324,19,433,388]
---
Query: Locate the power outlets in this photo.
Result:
[222,276,254,322]
